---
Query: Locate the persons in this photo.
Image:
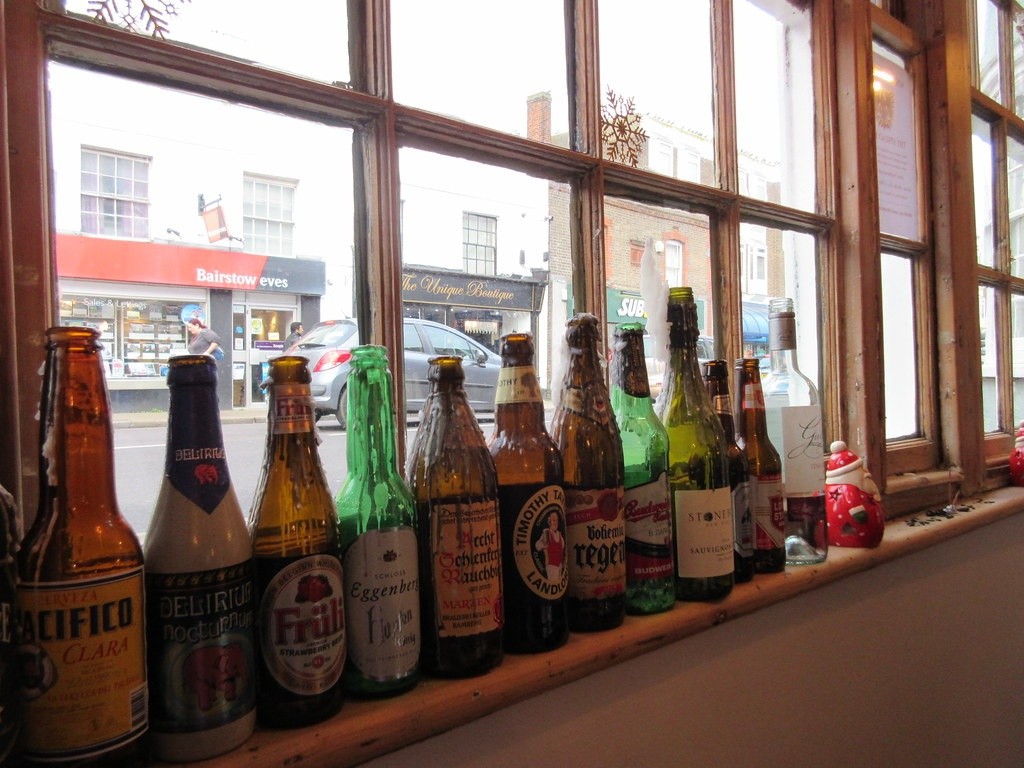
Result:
[187,319,220,355]
[282,322,303,352]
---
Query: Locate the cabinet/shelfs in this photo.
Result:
[121,301,189,377]
[61,296,117,371]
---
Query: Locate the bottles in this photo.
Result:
[761,296,830,564]
[729,356,787,573]
[405,356,506,676]
[656,288,736,602]
[247,356,347,727]
[702,358,759,584]
[552,315,625,634]
[485,333,570,653]
[14,325,148,768]
[333,343,421,697]
[609,323,676,616]
[146,354,258,761]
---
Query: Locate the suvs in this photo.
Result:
[278,317,503,429]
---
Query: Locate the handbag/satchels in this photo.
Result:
[213,346,225,360]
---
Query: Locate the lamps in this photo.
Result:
[229,235,245,244]
[656,241,664,252]
[166,227,184,240]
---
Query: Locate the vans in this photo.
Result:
[641,335,715,400]
